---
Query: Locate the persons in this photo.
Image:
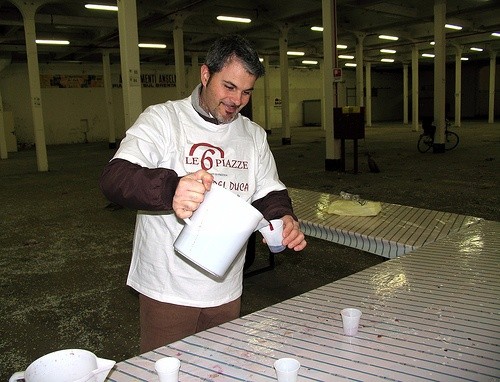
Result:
[101,34,306,355]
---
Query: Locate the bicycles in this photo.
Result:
[417,118,459,151]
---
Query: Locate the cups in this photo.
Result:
[154,357,180,382]
[258,219,286,253]
[340,308,362,336]
[274,358,300,382]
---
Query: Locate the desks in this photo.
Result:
[105,187,500,382]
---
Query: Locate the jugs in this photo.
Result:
[9,349,116,382]
[172,182,270,279]
